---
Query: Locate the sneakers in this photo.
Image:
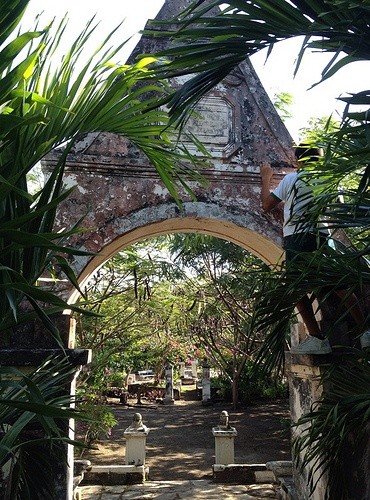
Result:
[290,334,333,354]
[360,329,370,350]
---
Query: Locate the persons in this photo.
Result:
[259,142,370,356]
